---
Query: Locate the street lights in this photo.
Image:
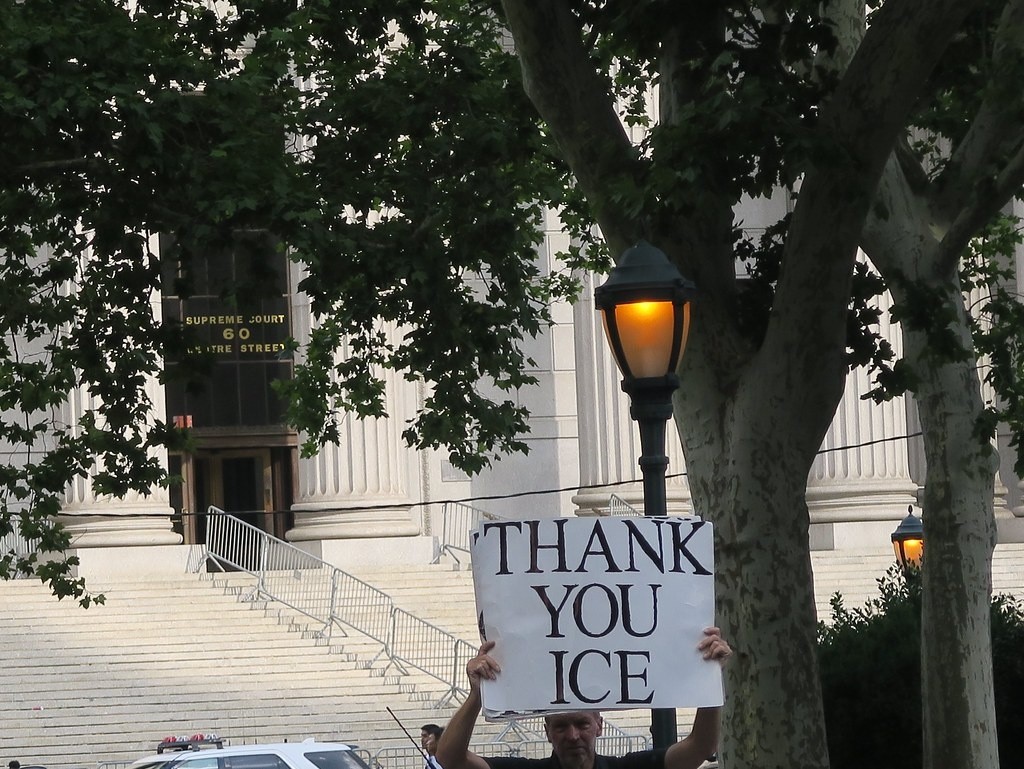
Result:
[593,232,697,753]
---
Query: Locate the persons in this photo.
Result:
[434,626,733,769]
[421,724,445,769]
[9,761,20,769]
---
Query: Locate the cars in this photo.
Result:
[122,736,376,769]
